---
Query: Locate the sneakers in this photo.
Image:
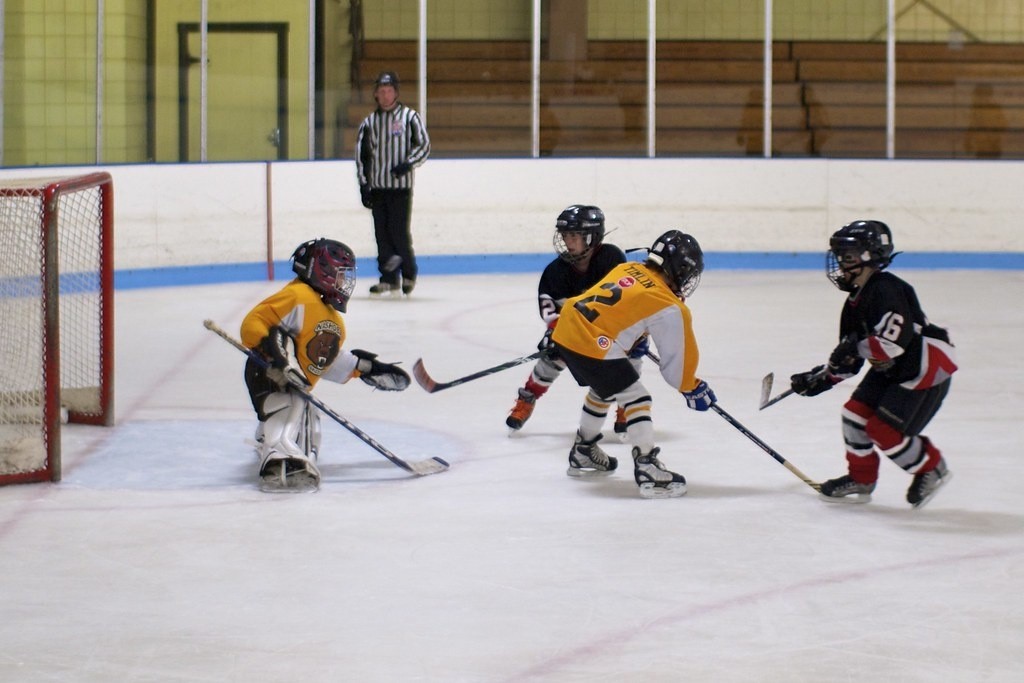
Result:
[506,387,536,438]
[567,428,618,476]
[403,268,418,297]
[370,279,400,295]
[906,456,951,509]
[614,405,630,444]
[631,445,687,498]
[818,473,877,503]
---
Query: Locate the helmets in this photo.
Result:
[827,219,895,293]
[377,72,398,86]
[647,230,704,298]
[292,238,357,314]
[553,205,605,265]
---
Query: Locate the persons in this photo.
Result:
[356,69,432,295]
[507,203,649,437]
[239,238,409,496]
[549,229,718,499]
[790,221,960,507]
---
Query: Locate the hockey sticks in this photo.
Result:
[645,350,824,494]
[413,346,555,394]
[759,355,856,411]
[203,317,451,477]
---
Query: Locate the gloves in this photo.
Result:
[360,184,373,209]
[630,337,650,358]
[352,350,410,392]
[537,318,562,362]
[827,333,865,375]
[790,364,836,396]
[390,163,411,179]
[682,380,717,412]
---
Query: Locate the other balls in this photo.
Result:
[384,255,403,272]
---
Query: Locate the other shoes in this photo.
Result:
[261,467,318,490]
